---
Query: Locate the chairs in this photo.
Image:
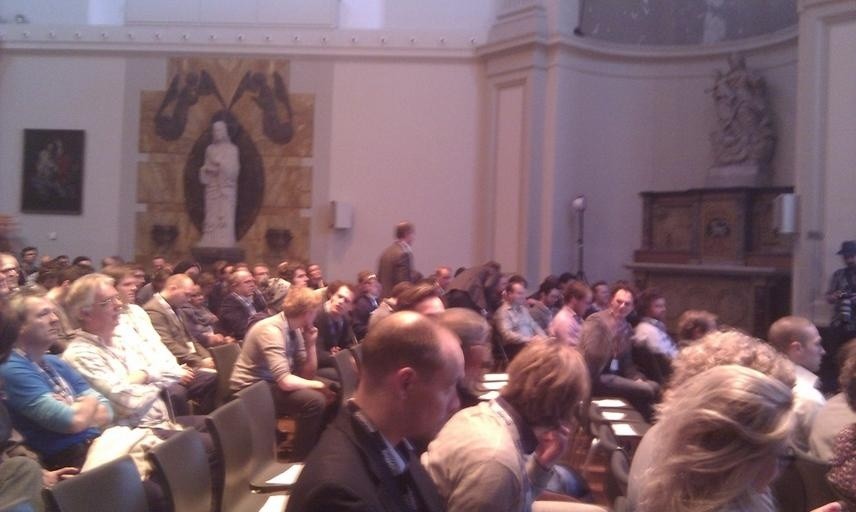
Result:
[0,337,856,511]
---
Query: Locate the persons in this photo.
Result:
[0,247,856,512]
[376,221,418,297]
[703,68,738,103]
[708,51,776,165]
[199,121,240,248]
[826,239,856,329]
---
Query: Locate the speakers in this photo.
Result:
[333,201,352,230]
[774,193,796,234]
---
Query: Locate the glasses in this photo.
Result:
[95,295,121,306]
[776,446,798,469]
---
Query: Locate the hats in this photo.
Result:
[836,240,856,257]
